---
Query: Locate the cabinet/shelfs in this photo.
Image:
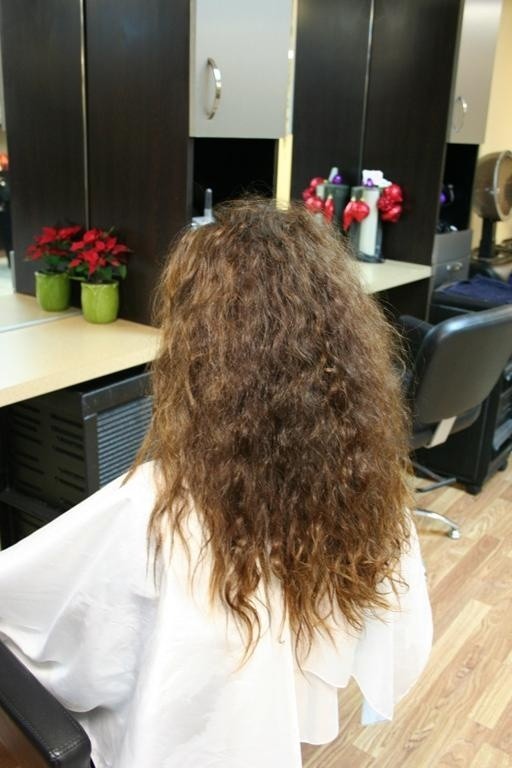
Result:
[433,230,473,289]
[188,1,296,145]
[445,1,502,146]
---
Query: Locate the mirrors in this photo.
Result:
[189,1,372,241]
[0,1,92,339]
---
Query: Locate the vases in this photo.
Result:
[78,279,122,323]
[36,270,69,313]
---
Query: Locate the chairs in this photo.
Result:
[391,303,511,539]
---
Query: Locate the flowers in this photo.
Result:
[24,225,78,273]
[65,224,131,287]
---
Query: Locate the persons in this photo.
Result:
[2,196,420,768]
